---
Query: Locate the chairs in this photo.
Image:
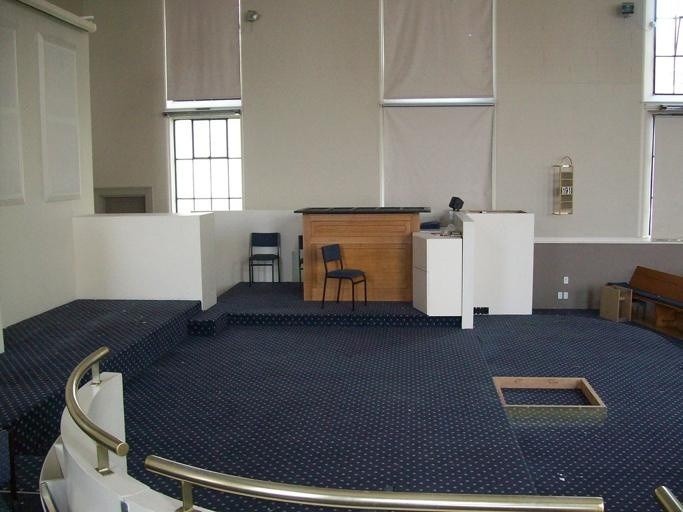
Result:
[297,235,303,284]
[321,243,366,311]
[248,233,280,287]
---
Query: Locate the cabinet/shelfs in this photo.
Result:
[412,227,461,317]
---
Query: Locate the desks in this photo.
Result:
[293,207,430,301]
